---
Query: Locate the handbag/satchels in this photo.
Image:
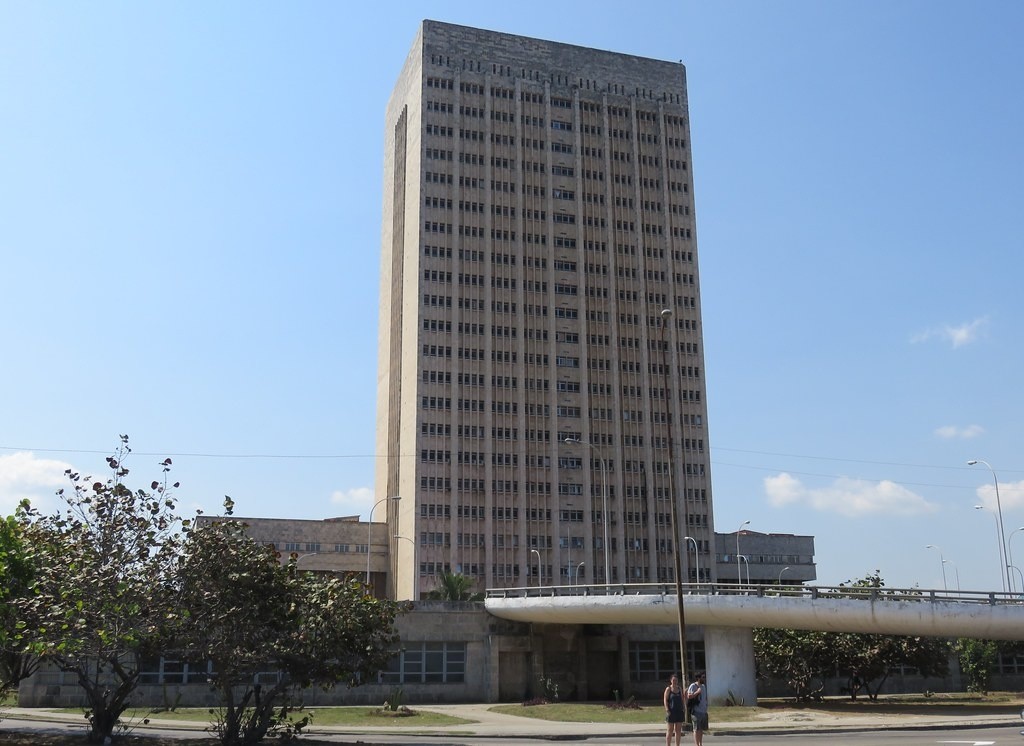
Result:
[688,683,701,707]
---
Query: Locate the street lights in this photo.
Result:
[966,459,1012,599]
[391,530,428,602]
[777,565,792,596]
[684,535,701,596]
[565,436,610,595]
[974,505,1007,598]
[1006,563,1024,596]
[1007,526,1024,595]
[574,560,585,597]
[531,549,546,596]
[734,520,749,595]
[736,552,753,594]
[362,495,403,598]
[926,544,949,598]
[941,557,961,599]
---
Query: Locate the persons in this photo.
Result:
[664,674,686,746]
[687,669,708,746]
